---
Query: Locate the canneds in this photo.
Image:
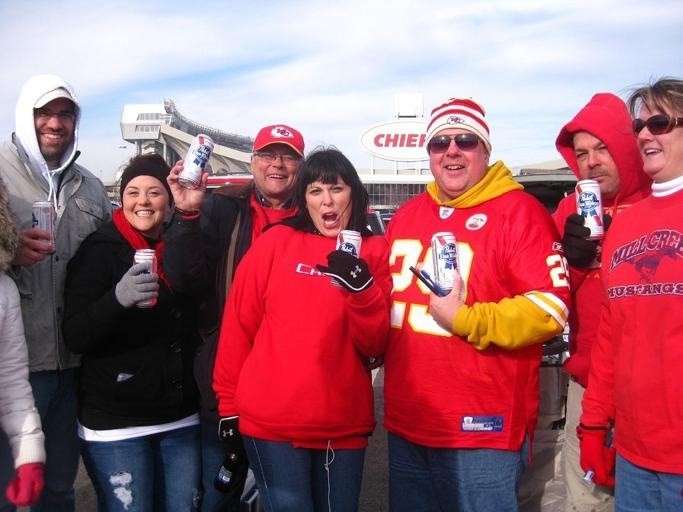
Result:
[575,180,605,241]
[431,231,460,291]
[177,134,214,189]
[32,201,55,254]
[133,248,157,309]
[331,229,362,287]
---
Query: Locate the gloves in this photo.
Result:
[6,460,46,506]
[316,249,374,293]
[217,415,245,463]
[563,214,612,268]
[115,262,160,308]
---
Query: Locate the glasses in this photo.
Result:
[428,133,480,154]
[254,150,299,161]
[36,109,76,121]
[632,113,682,135]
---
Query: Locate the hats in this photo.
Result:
[253,125,305,157]
[33,87,77,109]
[424,98,492,157]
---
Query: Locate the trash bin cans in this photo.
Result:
[538,335,568,427]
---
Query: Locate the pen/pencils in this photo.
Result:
[409,266,447,297]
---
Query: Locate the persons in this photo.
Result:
[386,99,572,511]
[157,123,306,507]
[211,144,392,512]
[0,178,47,508]
[60,155,202,509]
[550,93,649,512]
[574,76,683,511]
[0,66,115,510]
